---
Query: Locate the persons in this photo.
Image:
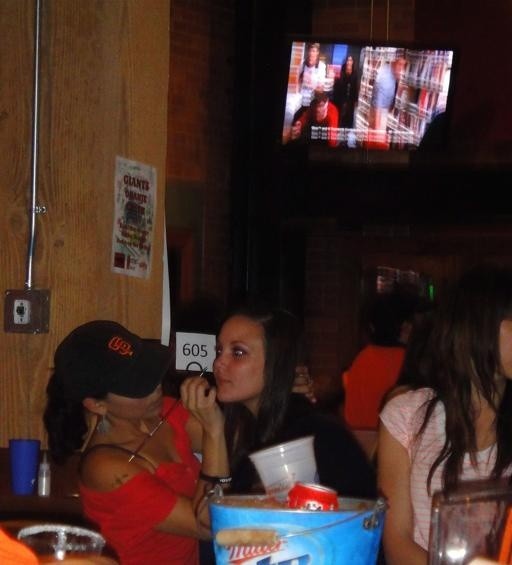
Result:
[335,288,428,429]
[377,300,512,564]
[216,297,369,495]
[283,44,409,145]
[46,322,320,565]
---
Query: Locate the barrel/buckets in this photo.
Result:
[195,486,389,565]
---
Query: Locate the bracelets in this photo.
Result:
[198,471,232,485]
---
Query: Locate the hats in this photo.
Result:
[56,321,170,398]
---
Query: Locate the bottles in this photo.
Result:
[37,451,51,498]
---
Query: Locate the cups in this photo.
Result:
[249,436,320,504]
[8,439,40,496]
[18,524,106,565]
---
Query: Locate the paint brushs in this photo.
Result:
[126,367,210,465]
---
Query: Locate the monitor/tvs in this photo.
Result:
[272,36,461,176]
